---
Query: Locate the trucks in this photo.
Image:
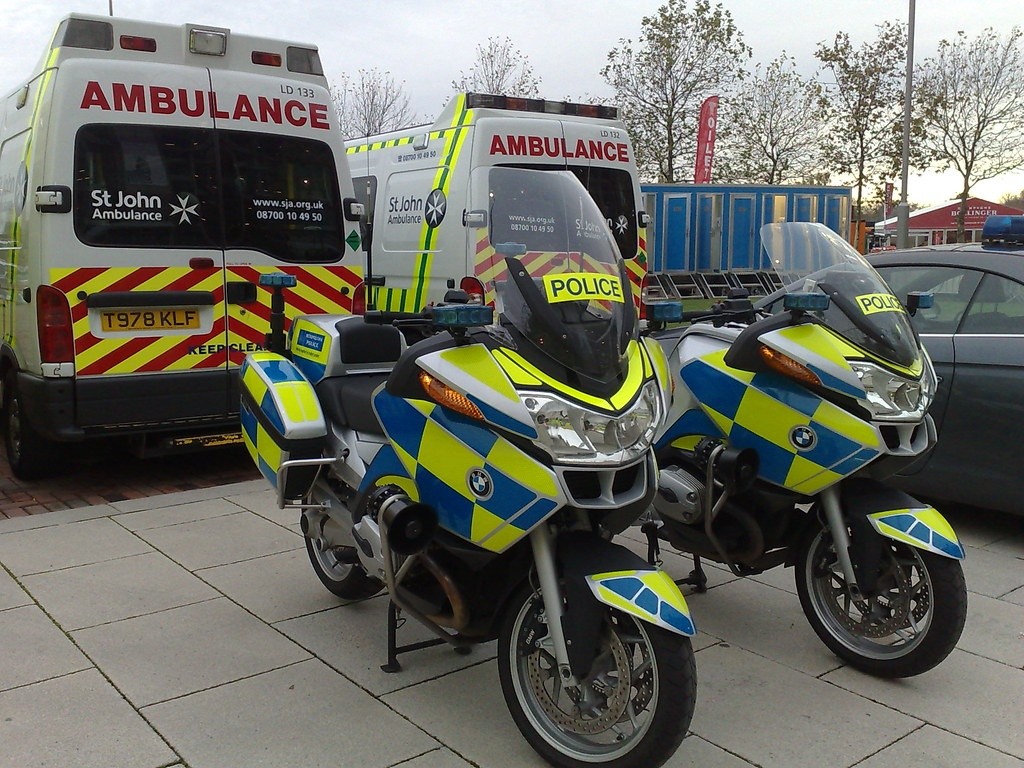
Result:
[639,182,853,329]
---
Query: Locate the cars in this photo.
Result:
[651,213,1023,530]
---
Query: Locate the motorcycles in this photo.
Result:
[625,221,967,682]
[238,162,700,768]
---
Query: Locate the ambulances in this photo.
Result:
[0,11,367,487]
[340,90,652,336]
